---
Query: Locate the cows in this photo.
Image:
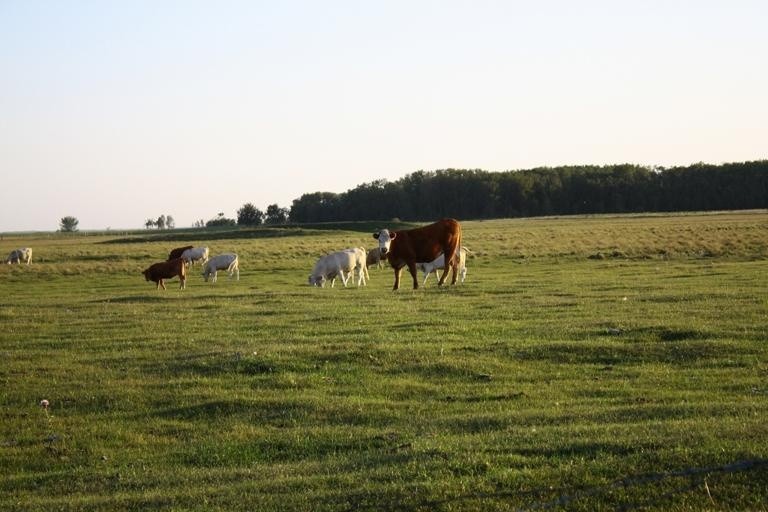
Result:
[141,244,240,291]
[307,217,479,291]
[4,247,33,266]
